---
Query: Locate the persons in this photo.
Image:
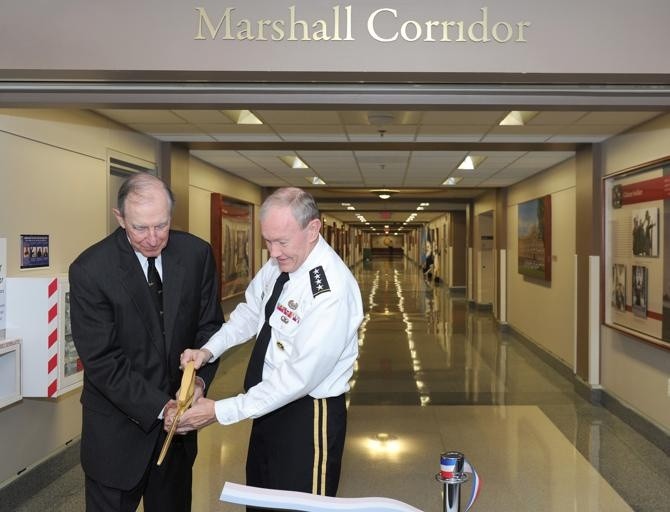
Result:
[68,172,225,511]
[167,186,365,511]
[616,216,657,310]
[24,247,48,258]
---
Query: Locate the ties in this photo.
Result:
[244,272,289,391]
[147,256,165,337]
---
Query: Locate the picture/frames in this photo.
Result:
[211,192,255,305]
[599,155,670,354]
